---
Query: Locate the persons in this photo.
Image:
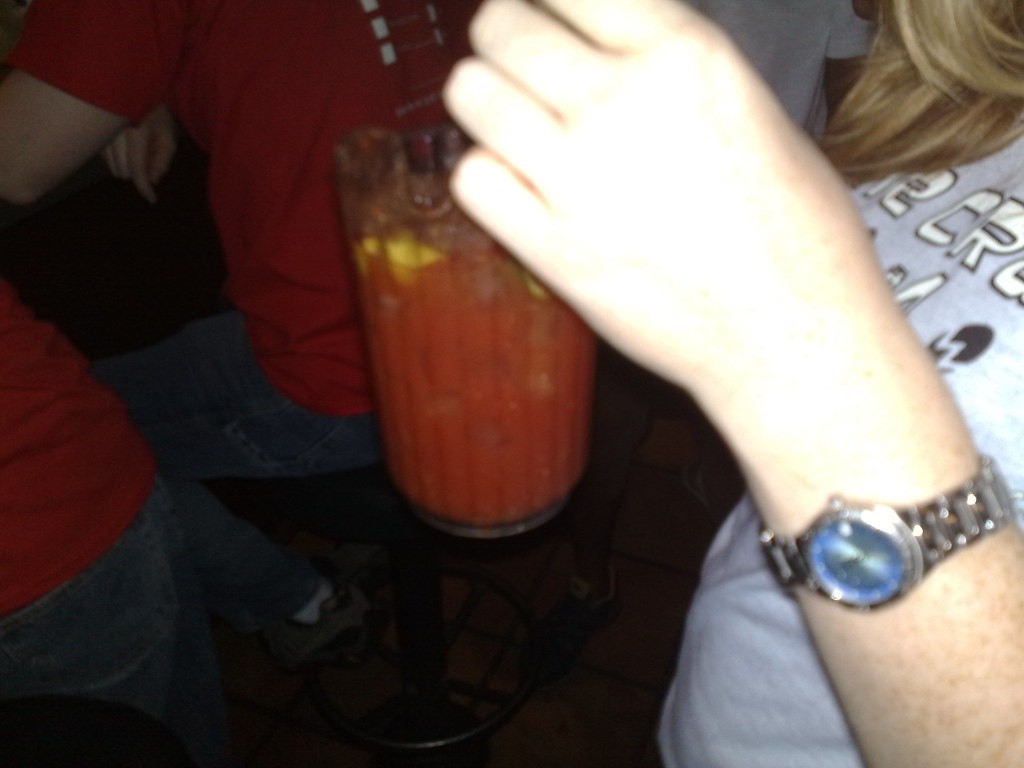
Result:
[445,1,1023,768]
[0,0,836,768]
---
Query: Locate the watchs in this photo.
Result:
[754,450,1014,616]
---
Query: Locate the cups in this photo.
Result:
[329,124,594,539]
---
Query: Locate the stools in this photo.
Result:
[288,476,546,750]
[0,695,198,768]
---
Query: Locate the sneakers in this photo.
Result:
[265,589,373,665]
[522,567,621,686]
